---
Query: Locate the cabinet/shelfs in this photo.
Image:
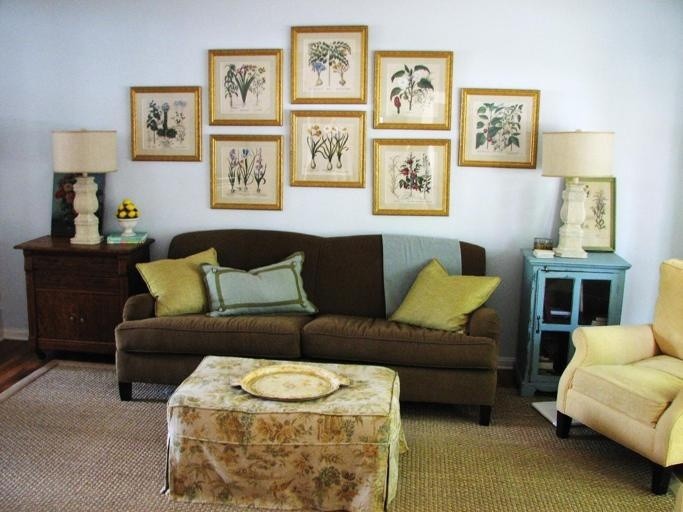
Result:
[11,232,155,361]
[511,247,632,399]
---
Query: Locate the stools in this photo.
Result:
[155,354,409,510]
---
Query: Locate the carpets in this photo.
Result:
[1,359,682,511]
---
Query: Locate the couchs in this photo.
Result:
[112,229,503,429]
[553,259,682,495]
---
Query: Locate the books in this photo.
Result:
[536,305,608,377]
[106,230,146,244]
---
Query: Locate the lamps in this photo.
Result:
[48,128,121,246]
[539,127,618,261]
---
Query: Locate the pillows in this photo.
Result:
[196,250,319,321]
[386,256,502,337]
[132,246,220,320]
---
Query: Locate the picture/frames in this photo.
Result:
[286,109,367,191]
[371,48,455,132]
[206,47,284,128]
[128,85,203,162]
[456,86,542,171]
[562,175,615,254]
[207,133,283,211]
[287,23,369,106]
[368,136,451,219]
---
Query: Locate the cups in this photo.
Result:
[532,238,552,251]
